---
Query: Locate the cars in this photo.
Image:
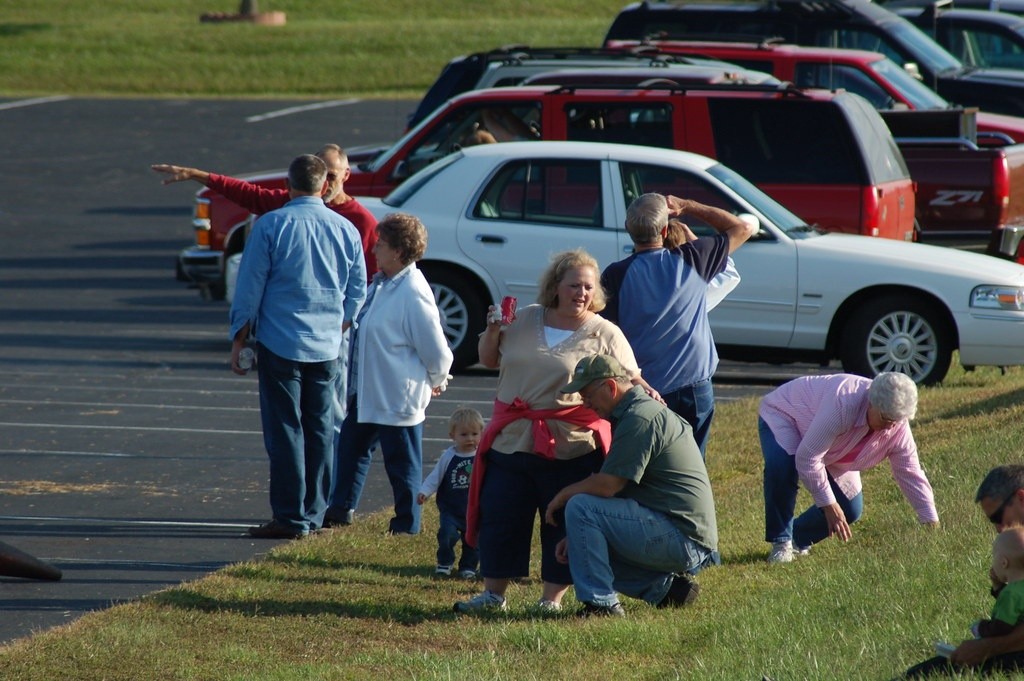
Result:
[229,139,1023,391]
[346,0,1024,255]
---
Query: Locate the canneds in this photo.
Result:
[498,295,517,326]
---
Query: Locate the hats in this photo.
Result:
[560,354,626,394]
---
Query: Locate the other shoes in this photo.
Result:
[436,562,453,576]
[459,569,474,581]
[322,517,349,528]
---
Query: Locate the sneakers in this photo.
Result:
[772,540,792,564]
[538,598,561,611]
[453,589,504,612]
[249,519,301,539]
[574,602,624,616]
[657,576,698,609]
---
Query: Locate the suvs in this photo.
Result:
[178,77,918,288]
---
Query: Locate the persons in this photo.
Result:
[151,143,385,504]
[595,190,754,461]
[227,155,368,538]
[415,407,484,579]
[757,371,939,566]
[902,463,1024,681]
[544,352,720,619]
[467,130,498,145]
[449,250,668,612]
[323,212,454,532]
[662,220,741,313]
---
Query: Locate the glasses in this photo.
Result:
[878,406,902,424]
[990,488,1022,524]
[580,378,618,400]
